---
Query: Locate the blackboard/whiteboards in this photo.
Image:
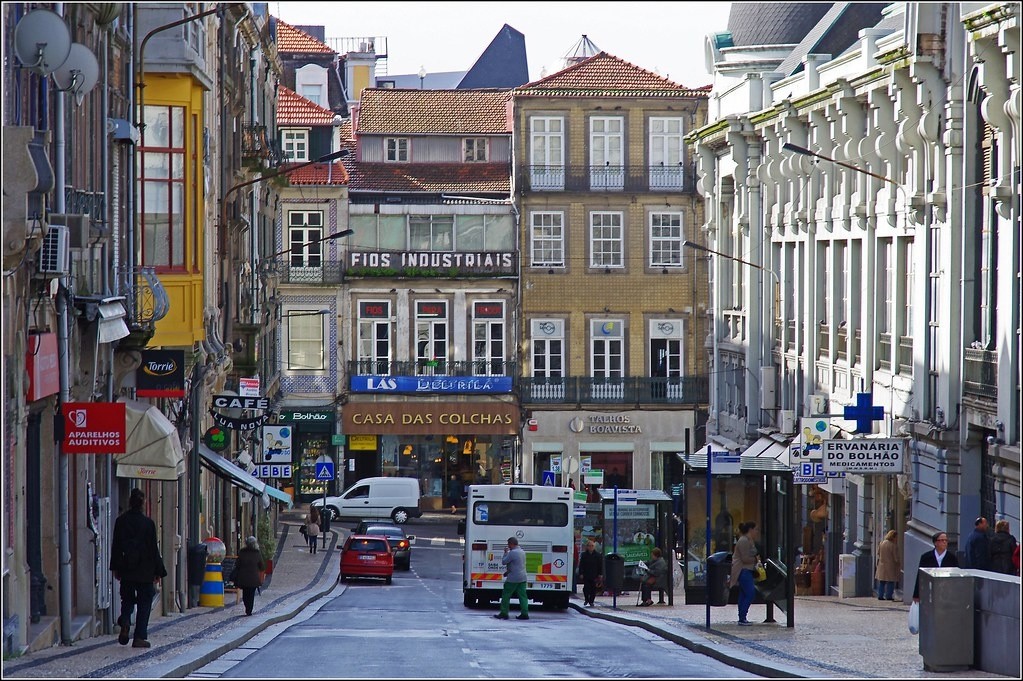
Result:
[221,556,241,591]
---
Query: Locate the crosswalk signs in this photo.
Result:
[542,472,555,486]
[315,463,334,481]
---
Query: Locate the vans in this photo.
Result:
[309,477,423,524]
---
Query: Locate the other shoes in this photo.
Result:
[516,613,530,620]
[132,641,151,648]
[118,624,129,645]
[589,601,594,606]
[637,600,654,607]
[494,612,509,620]
[878,596,885,600]
[584,600,588,605]
[739,619,752,625]
[886,597,895,601]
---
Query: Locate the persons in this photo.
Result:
[964,517,1017,575]
[447,475,462,514]
[640,548,668,606]
[308,518,319,554]
[493,537,530,620]
[305,506,321,548]
[731,521,760,625]
[573,535,603,607]
[636,534,650,546]
[875,530,900,600]
[234,536,265,616]
[109,488,167,647]
[913,532,961,604]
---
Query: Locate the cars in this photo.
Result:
[351,519,395,535]
[364,526,414,571]
[337,534,393,584]
[687,552,705,572]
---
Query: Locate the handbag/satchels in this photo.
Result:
[640,573,656,585]
[755,561,766,582]
[908,601,919,635]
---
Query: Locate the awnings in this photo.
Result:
[199,443,294,513]
[115,396,186,481]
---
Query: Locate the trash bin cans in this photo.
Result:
[320,508,332,532]
[707,551,732,606]
[604,553,625,593]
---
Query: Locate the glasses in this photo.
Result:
[936,539,947,541]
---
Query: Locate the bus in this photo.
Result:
[458,483,574,610]
